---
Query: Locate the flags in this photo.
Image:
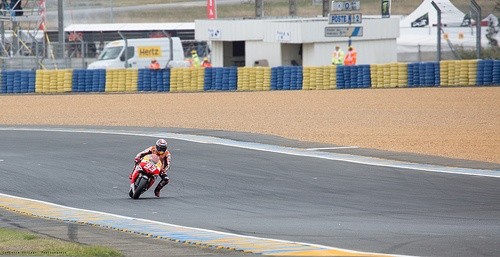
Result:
[39,22,46,30]
[381,0,389,18]
[38,0,45,21]
[207,0,216,20]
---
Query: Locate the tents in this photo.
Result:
[400,0,476,27]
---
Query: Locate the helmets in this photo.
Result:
[155,139,168,154]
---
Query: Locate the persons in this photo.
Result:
[128,139,171,197]
[331,45,344,64]
[200,57,213,67]
[343,46,356,66]
[148,58,160,68]
[190,49,201,67]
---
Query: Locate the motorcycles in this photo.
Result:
[127,154,164,199]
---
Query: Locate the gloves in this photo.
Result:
[134,158,141,164]
[160,168,166,175]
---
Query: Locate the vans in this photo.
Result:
[87,36,185,71]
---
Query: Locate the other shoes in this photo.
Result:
[154,189,160,197]
